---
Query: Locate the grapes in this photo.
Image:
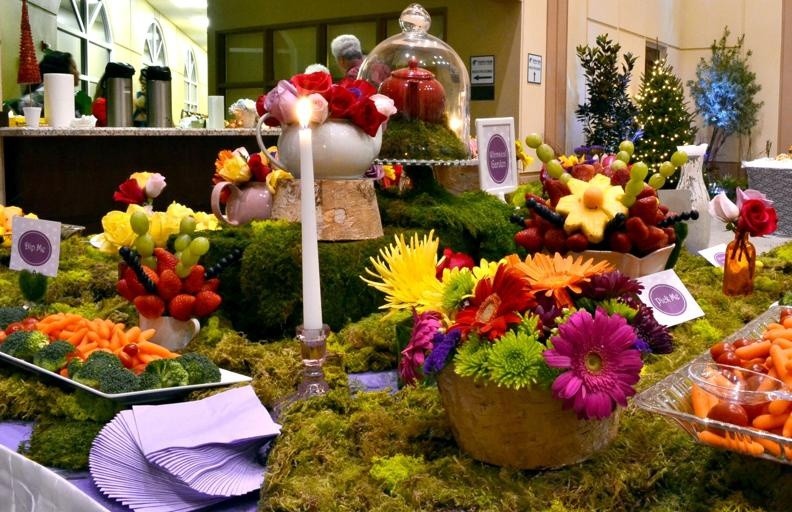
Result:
[526,132,573,185]
[130,210,211,279]
[613,140,686,204]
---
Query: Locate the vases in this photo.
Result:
[674,143,711,256]
[142,205,152,215]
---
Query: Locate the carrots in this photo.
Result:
[35,312,182,377]
[689,309,792,462]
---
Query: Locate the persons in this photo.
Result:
[17,51,92,119]
[134,69,148,127]
[331,34,391,88]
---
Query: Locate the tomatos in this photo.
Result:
[0,317,37,343]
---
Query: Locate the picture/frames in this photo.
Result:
[470,55,495,85]
[527,53,542,84]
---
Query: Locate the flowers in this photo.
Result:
[113,171,167,206]
[358,228,675,421]
[211,145,277,205]
[707,188,779,262]
[256,62,398,137]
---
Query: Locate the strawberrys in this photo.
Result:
[513,167,673,252]
[117,247,221,320]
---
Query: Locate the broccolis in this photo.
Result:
[0,305,222,393]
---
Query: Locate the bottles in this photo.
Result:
[141,65,174,128]
[103,62,135,127]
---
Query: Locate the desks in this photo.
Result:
[0,126,283,238]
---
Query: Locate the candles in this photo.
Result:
[294,96,322,331]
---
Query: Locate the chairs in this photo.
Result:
[745,166,792,238]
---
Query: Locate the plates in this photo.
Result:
[629,302,792,465]
[0,321,255,399]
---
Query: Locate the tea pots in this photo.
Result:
[380,57,447,124]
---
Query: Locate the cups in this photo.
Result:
[207,94,225,130]
[23,106,41,128]
[42,72,76,128]
[136,311,201,354]
